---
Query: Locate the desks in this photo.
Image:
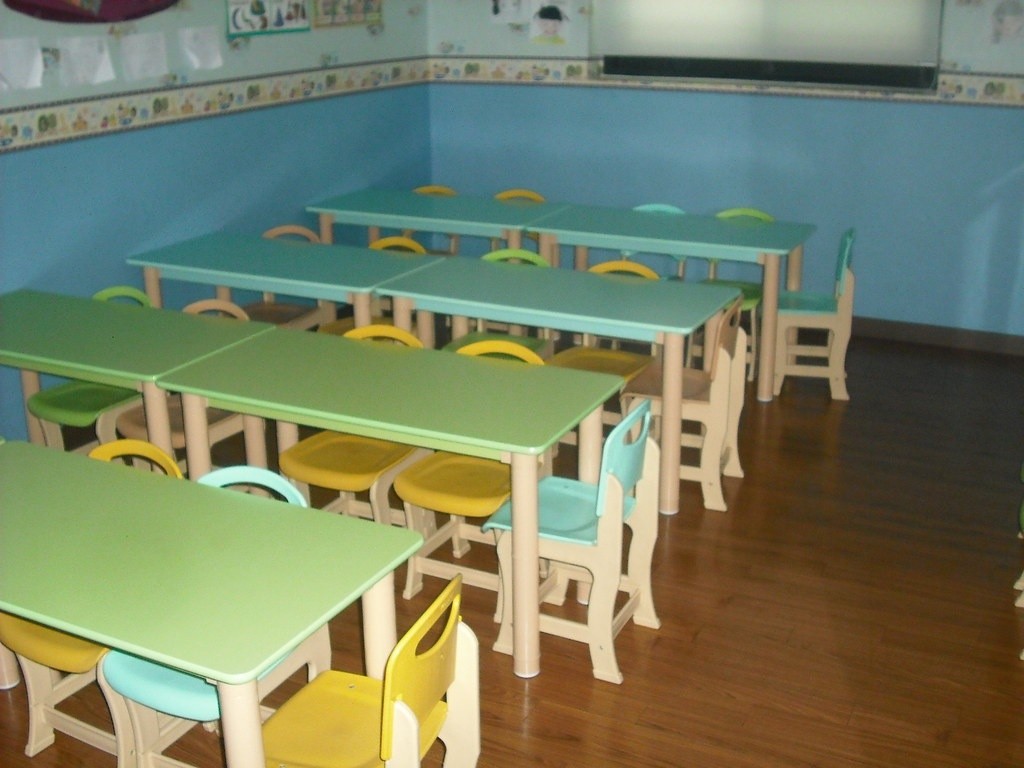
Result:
[0,439,424,768]
[124,228,448,328]
[304,187,575,336]
[155,325,627,679]
[375,253,743,515]
[0,289,276,476]
[528,207,817,401]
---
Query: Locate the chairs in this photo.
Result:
[1,188,856,768]
